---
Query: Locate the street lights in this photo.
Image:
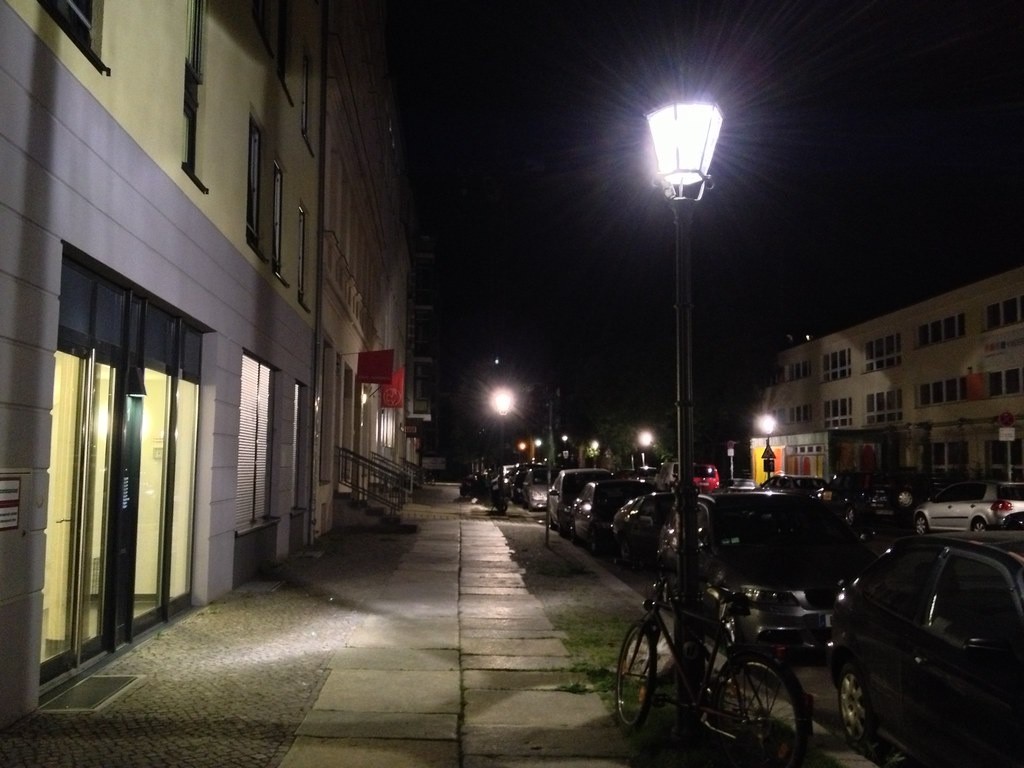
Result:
[756,413,779,480]
[489,386,513,514]
[642,72,725,752]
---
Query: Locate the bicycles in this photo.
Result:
[615,559,814,768]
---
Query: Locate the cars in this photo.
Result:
[823,529,1024,768]
[912,479,1024,536]
[655,488,882,660]
[484,459,919,572]
